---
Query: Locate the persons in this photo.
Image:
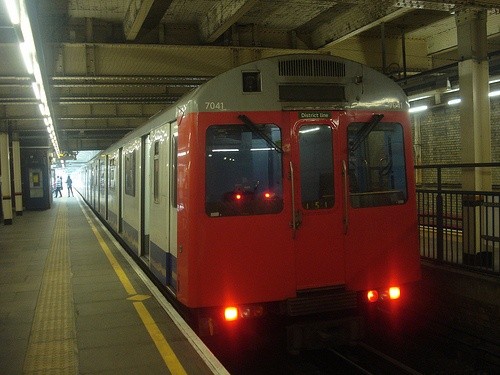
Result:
[54,176,64,197]
[66,176,74,197]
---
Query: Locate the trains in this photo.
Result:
[70,53,422,355]
